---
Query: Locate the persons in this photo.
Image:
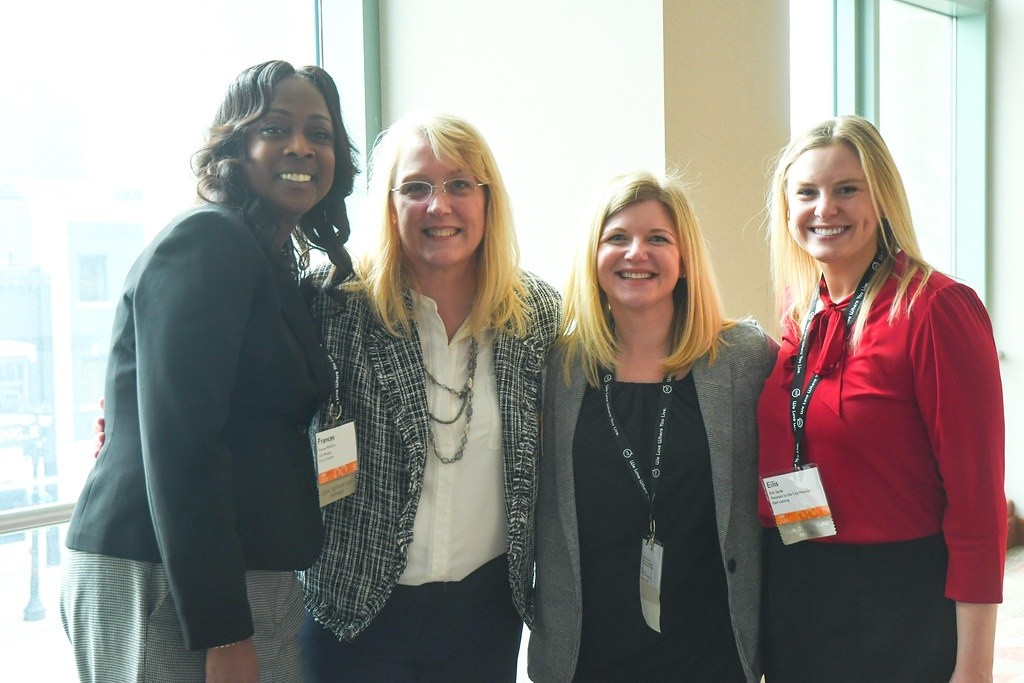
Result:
[59,57,365,683]
[526,175,789,682]
[746,113,1008,682]
[93,115,566,683]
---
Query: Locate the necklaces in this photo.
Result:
[411,329,479,468]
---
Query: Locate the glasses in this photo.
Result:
[391,178,487,203]
